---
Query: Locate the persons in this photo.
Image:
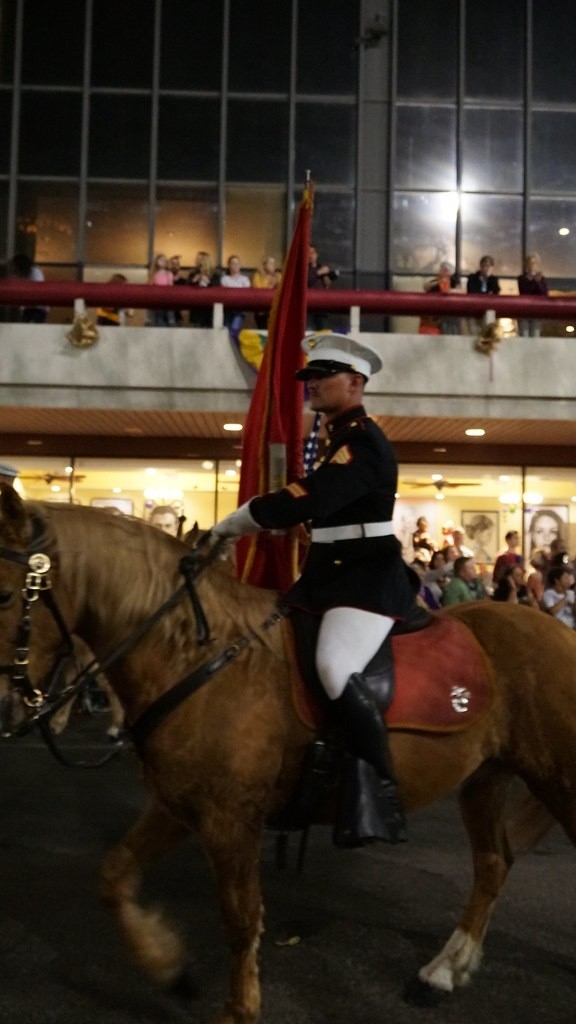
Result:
[93,273,133,327]
[189,252,211,327]
[466,256,501,330]
[308,243,332,330]
[529,509,568,565]
[9,253,52,323]
[220,256,252,330]
[423,262,462,335]
[219,329,422,849]
[149,505,180,540]
[465,514,495,563]
[251,255,283,329]
[144,254,190,328]
[517,251,550,336]
[406,516,576,627]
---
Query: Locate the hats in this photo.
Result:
[294,333,383,382]
[414,547,432,563]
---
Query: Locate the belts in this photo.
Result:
[311,520,394,544]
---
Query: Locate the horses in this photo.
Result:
[0,479,576,1024]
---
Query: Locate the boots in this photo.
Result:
[316,672,409,849]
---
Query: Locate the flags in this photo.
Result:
[237,179,312,595]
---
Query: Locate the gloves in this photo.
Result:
[209,495,265,551]
